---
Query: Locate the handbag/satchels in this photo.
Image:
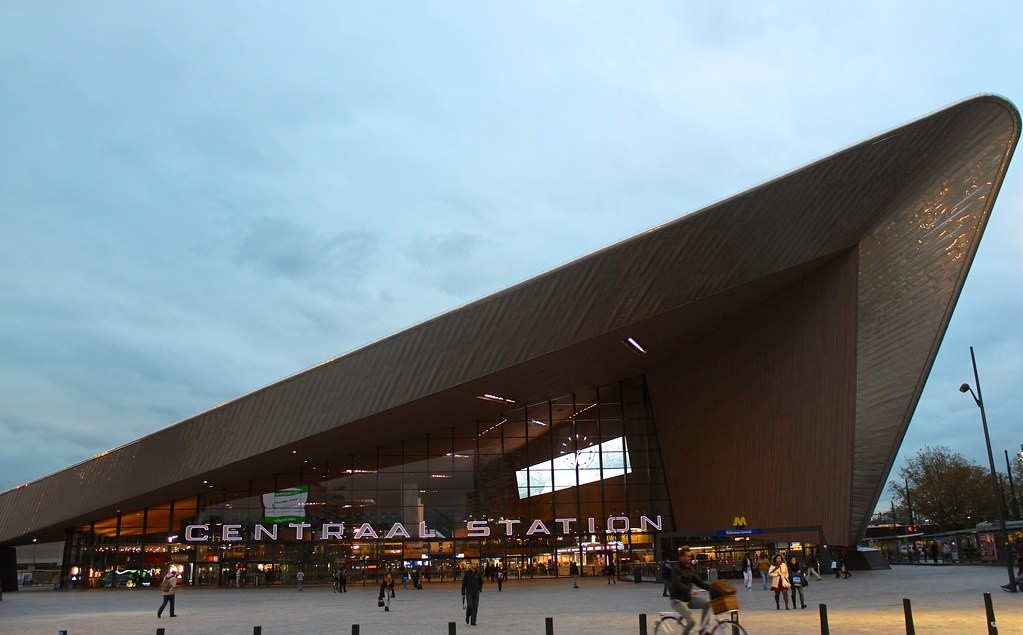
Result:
[782,578,791,587]
[378,599,384,607]
[801,577,808,587]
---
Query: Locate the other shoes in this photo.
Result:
[471,622,477,626]
[158,612,160,618]
[339,590,342,593]
[465,616,469,624]
[777,607,780,609]
[573,585,579,588]
[786,607,789,609]
[170,615,176,617]
[794,605,796,609]
[801,605,807,609]
[343,589,347,593]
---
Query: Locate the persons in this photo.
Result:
[741,553,753,592]
[841,549,853,579]
[332,564,347,593]
[942,542,951,564]
[788,553,808,610]
[378,572,395,612]
[930,541,939,563]
[832,550,842,578]
[922,543,929,562]
[156,570,178,618]
[401,561,547,590]
[495,567,504,592]
[1001,528,1023,593]
[754,553,770,590]
[804,549,821,581]
[768,553,791,610]
[661,545,712,635]
[227,567,242,588]
[264,568,272,589]
[461,559,483,626]
[571,562,580,588]
[297,568,304,592]
[607,561,616,584]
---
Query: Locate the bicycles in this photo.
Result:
[652,589,748,635]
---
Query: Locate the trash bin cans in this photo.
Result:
[952,550,958,563]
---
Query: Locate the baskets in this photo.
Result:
[711,595,740,615]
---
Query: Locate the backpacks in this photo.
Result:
[498,572,503,579]
[161,576,174,592]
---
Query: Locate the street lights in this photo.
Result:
[959,383,1017,592]
